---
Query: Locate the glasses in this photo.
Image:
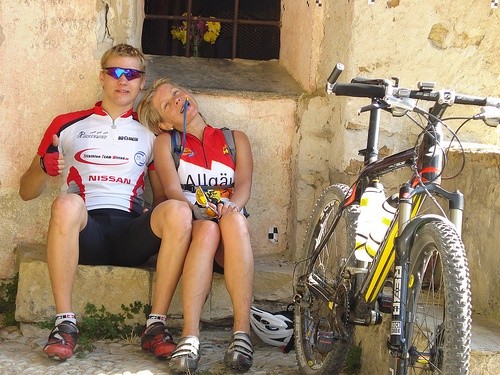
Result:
[100,67,144,81]
[195,186,220,218]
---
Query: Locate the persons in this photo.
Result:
[136,76,256,375]
[18,44,193,362]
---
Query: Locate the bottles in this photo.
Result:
[354,178,385,263]
[365,193,400,256]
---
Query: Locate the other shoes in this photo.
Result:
[168,335,201,375]
[224,331,254,373]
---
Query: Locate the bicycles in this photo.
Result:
[291,60,500,375]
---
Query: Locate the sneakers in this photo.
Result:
[141,322,177,358]
[43,320,82,360]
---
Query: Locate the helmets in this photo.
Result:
[249,306,295,349]
